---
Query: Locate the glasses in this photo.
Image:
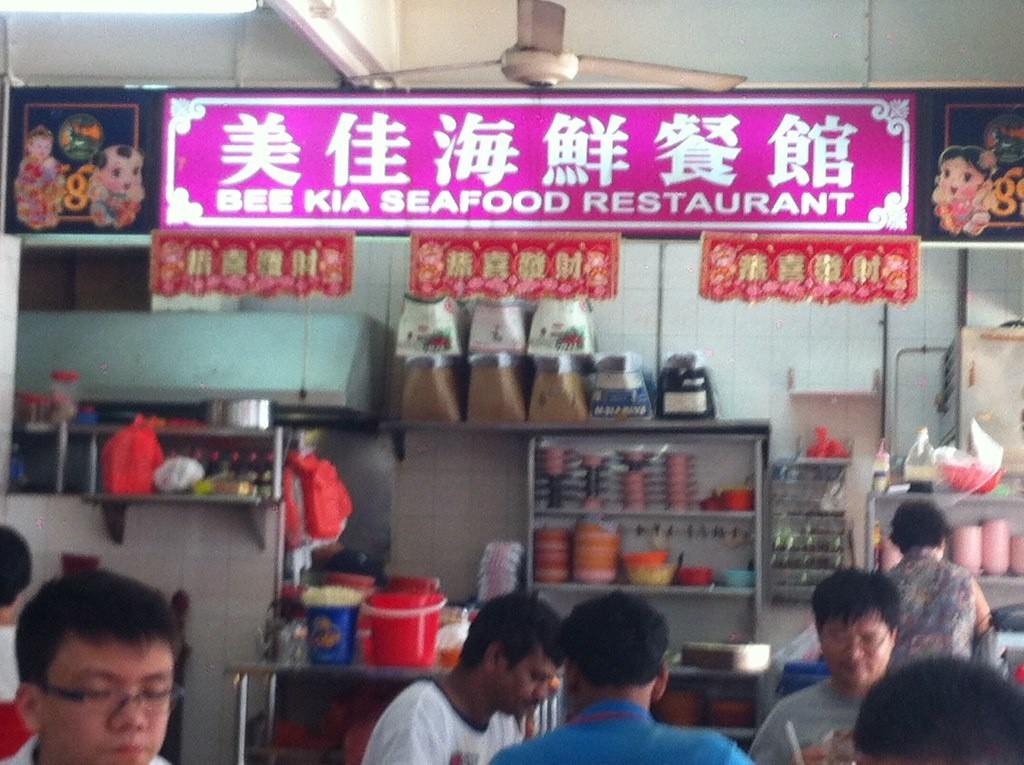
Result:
[31,682,185,716]
[821,626,895,650]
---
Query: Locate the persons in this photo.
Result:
[359,592,565,765]
[0,569,183,765]
[854,657,1024,765]
[0,523,36,760]
[487,591,753,765]
[881,499,991,672]
[749,568,902,765]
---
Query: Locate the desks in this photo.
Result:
[226,653,461,765]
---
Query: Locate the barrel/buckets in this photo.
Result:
[205,397,279,428]
[361,594,449,667]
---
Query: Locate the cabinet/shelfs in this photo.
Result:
[526,436,766,740]
[5,420,284,505]
[865,490,1024,648]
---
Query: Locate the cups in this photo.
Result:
[302,602,360,666]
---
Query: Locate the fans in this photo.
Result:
[334,0,748,96]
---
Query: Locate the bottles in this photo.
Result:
[167,448,273,495]
[903,427,936,494]
[872,449,892,492]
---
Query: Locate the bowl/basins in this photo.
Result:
[534,447,755,511]
[533,523,755,587]
[880,538,905,570]
[953,519,1024,577]
[945,464,1006,494]
